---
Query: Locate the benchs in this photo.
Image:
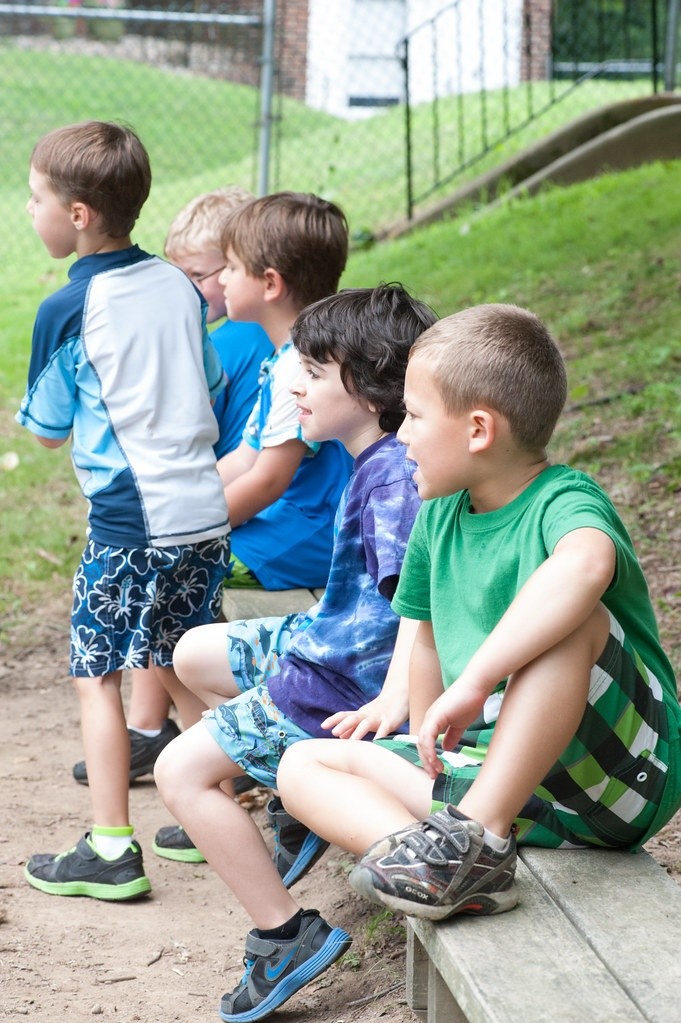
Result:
[221,584,681,1022]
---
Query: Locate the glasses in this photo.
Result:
[190,265,223,289]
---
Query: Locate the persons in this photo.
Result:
[15,122,233,902]
[276,303,681,919]
[163,188,351,590]
[152,286,440,1023]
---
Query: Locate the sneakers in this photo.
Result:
[23,830,152,902]
[218,908,352,1022]
[152,826,209,863]
[263,797,332,889]
[348,805,519,921]
[71,719,182,786]
